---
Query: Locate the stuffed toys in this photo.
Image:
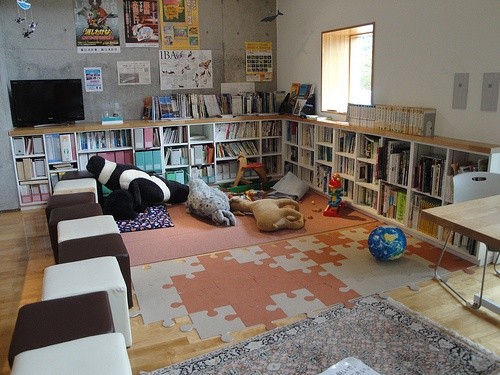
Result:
[185,178,235,227]
[229,196,305,232]
[86,156,189,220]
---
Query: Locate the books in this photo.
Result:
[285,122,487,256]
[163,120,282,176]
[348,102,436,136]
[12,121,162,204]
[142,83,314,121]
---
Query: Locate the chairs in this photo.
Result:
[434,172,500,310]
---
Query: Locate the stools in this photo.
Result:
[58,233,133,308]
[49,203,103,263]
[57,215,119,244]
[42,256,132,348]
[10,332,133,375]
[45,192,96,224]
[54,178,98,203]
[61,172,102,202]
[7,291,114,368]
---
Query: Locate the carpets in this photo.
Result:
[140,292,500,375]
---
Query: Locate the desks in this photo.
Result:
[421,195,500,315]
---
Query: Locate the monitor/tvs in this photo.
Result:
[10,79,85,126]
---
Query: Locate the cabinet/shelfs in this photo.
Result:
[8,118,499,268]
